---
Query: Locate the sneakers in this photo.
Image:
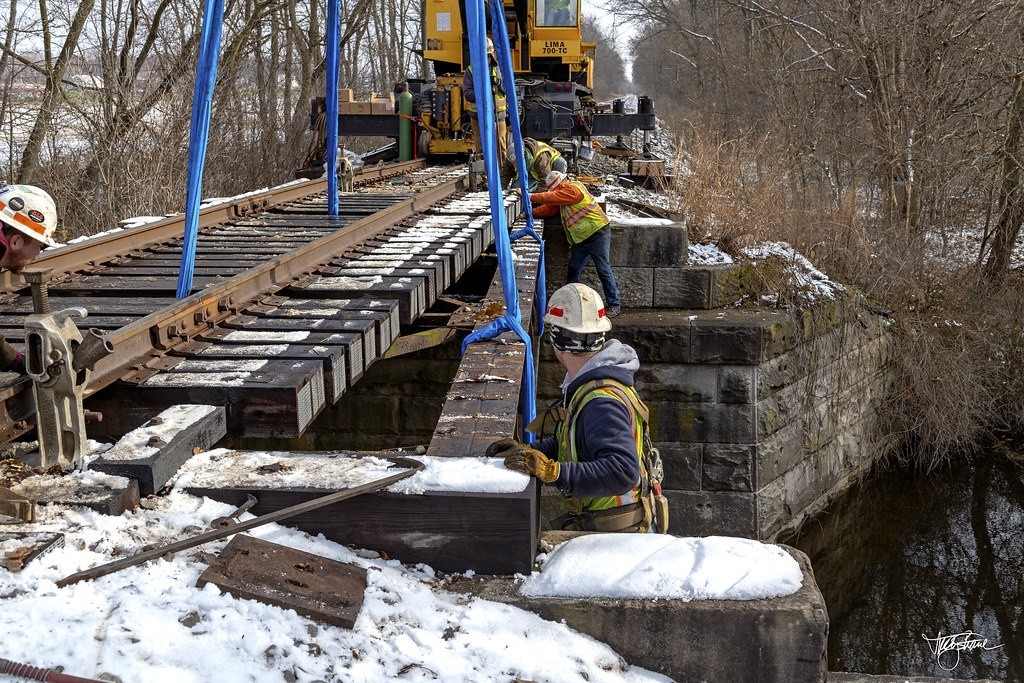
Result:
[606,305,621,318]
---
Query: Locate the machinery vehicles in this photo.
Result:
[309,0,656,187]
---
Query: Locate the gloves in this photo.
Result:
[508,188,522,196]
[492,76,499,94]
[517,213,526,219]
[485,438,561,484]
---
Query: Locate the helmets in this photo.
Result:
[487,36,495,54]
[0,184,58,248]
[506,143,516,163]
[545,171,568,191]
[542,282,613,334]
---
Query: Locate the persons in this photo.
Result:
[499,136,568,194]
[518,169,621,318]
[463,37,507,192]
[545,0,571,25]
[0,183,61,374]
[484,284,668,534]
